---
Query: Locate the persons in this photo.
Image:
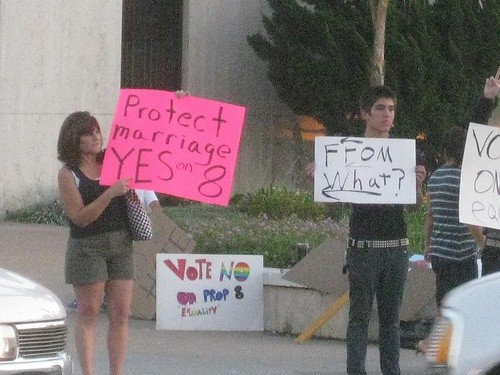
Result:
[304,85,427,375]
[56,89,191,374]
[71,189,161,314]
[464,75,500,275]
[422,126,487,310]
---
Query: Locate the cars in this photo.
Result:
[423,271,500,375]
[0,268,69,375]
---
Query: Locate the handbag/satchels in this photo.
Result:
[121,189,152,241]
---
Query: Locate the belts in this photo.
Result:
[346,237,411,249]
